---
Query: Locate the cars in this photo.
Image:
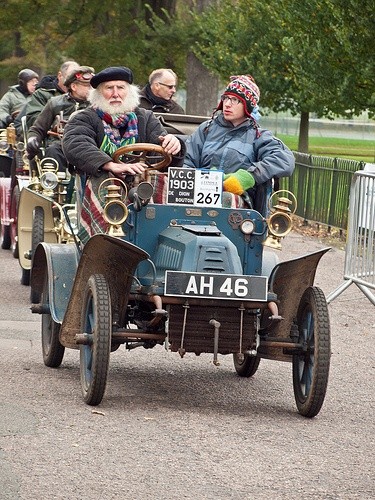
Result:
[19,142,333,418]
[0,116,74,285]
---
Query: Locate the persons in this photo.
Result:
[14,61,79,142]
[183,75,294,208]
[138,68,185,115]
[61,66,187,175]
[28,68,92,160]
[0,68,38,125]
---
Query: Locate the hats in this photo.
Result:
[89,66,133,89]
[18,68,39,91]
[63,66,95,86]
[218,74,260,117]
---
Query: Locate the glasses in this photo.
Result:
[76,73,95,81]
[221,94,243,105]
[157,82,176,89]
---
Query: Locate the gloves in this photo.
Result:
[6,116,13,126]
[223,169,255,195]
[27,137,42,155]
